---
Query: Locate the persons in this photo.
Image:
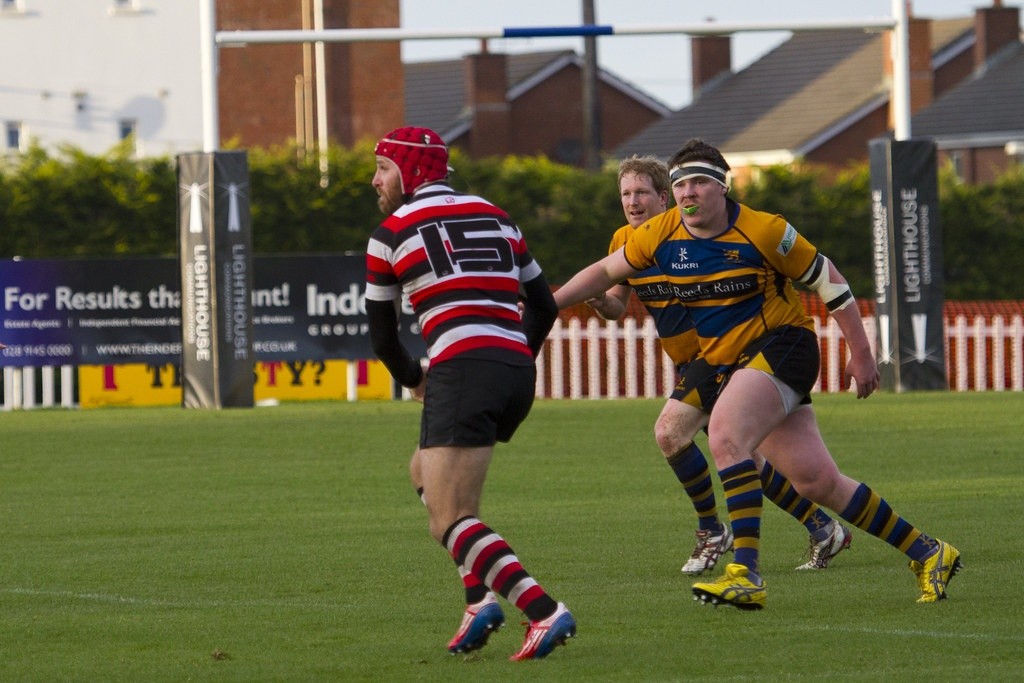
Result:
[364,125,576,661]
[519,138,960,611]
[582,153,855,575]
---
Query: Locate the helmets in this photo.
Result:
[374,127,449,195]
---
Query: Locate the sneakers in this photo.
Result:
[513,601,579,663]
[693,563,769,610]
[794,520,854,571]
[908,538,961,604]
[680,524,735,574]
[444,592,506,656]
